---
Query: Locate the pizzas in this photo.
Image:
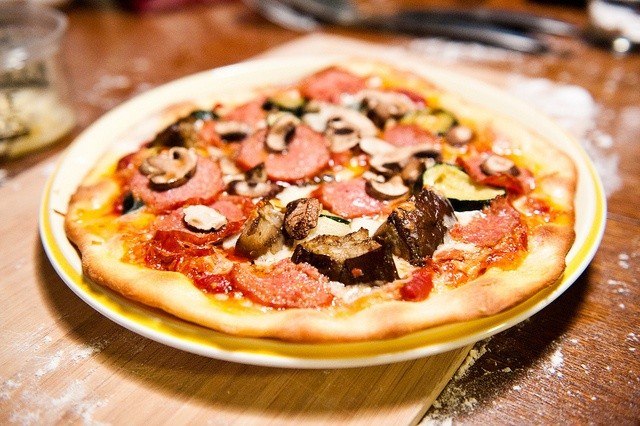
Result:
[64,57,578,343]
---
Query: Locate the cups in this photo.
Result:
[1,8,77,156]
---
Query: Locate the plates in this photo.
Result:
[37,55,608,370]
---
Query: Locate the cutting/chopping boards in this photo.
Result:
[0,32,591,426]
